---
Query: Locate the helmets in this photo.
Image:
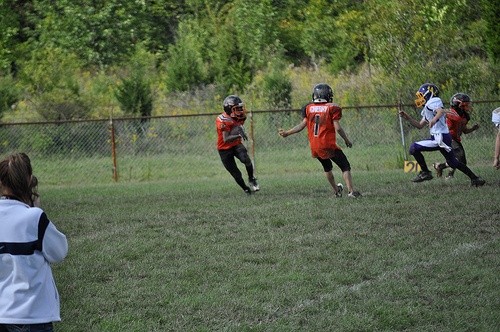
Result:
[223,95,246,121]
[312,84,333,103]
[449,92,472,113]
[414,83,440,107]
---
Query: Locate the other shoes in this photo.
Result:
[335,183,343,197]
[471,177,487,186]
[446,171,454,179]
[245,177,260,193]
[411,171,433,183]
[433,162,442,177]
[348,191,357,199]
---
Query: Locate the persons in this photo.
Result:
[492,107,500,169]
[399,83,486,187]
[433,93,480,178]
[0,152,68,332]
[279,84,362,198]
[215,95,260,197]
